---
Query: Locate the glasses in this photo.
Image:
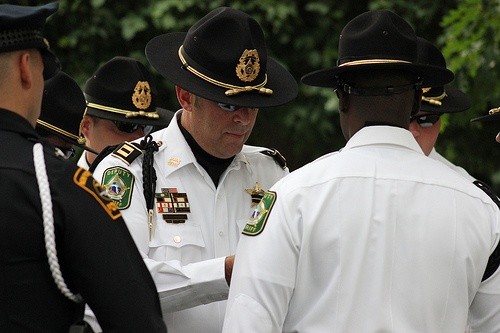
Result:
[54,145,75,160]
[110,119,147,133]
[217,102,261,112]
[409,113,443,128]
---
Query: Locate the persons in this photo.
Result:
[0,0,174,333]
[222,8,500,333]
[84,9,291,333]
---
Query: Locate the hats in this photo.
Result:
[145,7,299,107]
[0,2,62,82]
[470,106,500,122]
[83,57,162,125]
[301,9,454,88]
[412,36,471,113]
[36,71,99,155]
